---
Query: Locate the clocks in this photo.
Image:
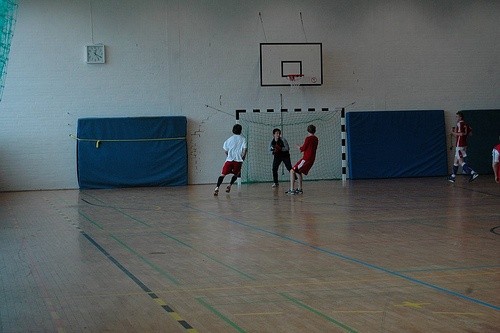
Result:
[86,45,106,64]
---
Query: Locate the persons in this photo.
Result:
[492,144,500,183]
[269,128,299,187]
[447,111,478,182]
[285,124,319,195]
[213,124,248,196]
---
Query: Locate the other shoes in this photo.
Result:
[469,172,479,182]
[448,176,455,183]
[272,183,279,188]
[226,184,231,193]
[285,188,295,195]
[214,187,219,196]
[296,187,303,194]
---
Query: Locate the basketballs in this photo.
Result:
[274,143,283,151]
